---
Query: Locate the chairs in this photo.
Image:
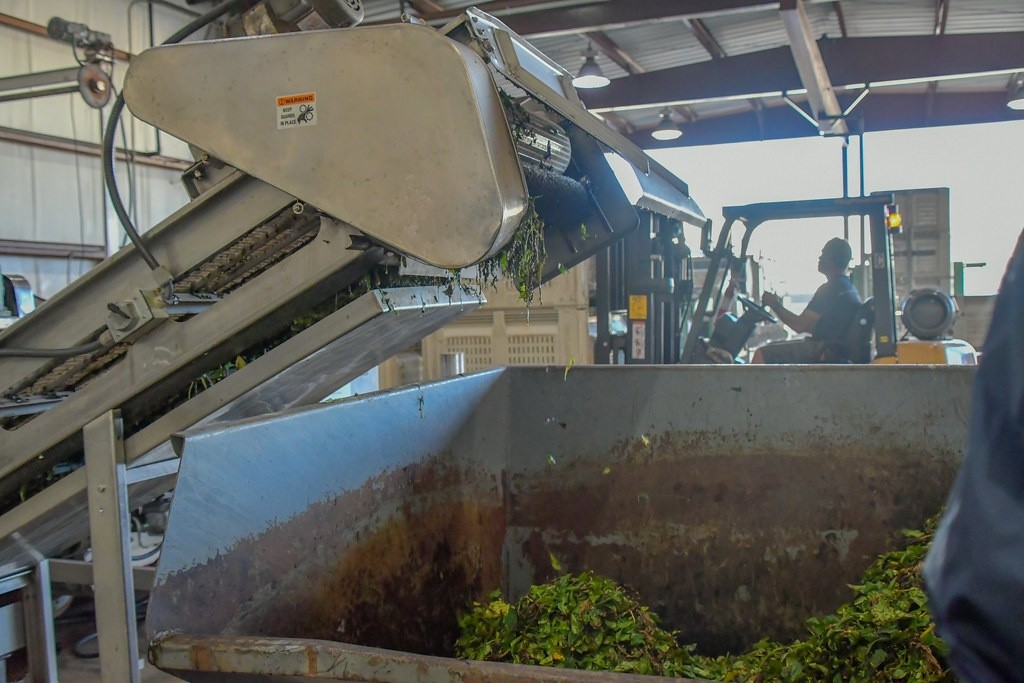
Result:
[858,297,876,362]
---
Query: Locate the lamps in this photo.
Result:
[651,107,683,140]
[572,44,611,89]
[887,205,904,233]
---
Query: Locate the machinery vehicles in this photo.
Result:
[595,192,980,364]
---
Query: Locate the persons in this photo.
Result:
[751,238,863,362]
